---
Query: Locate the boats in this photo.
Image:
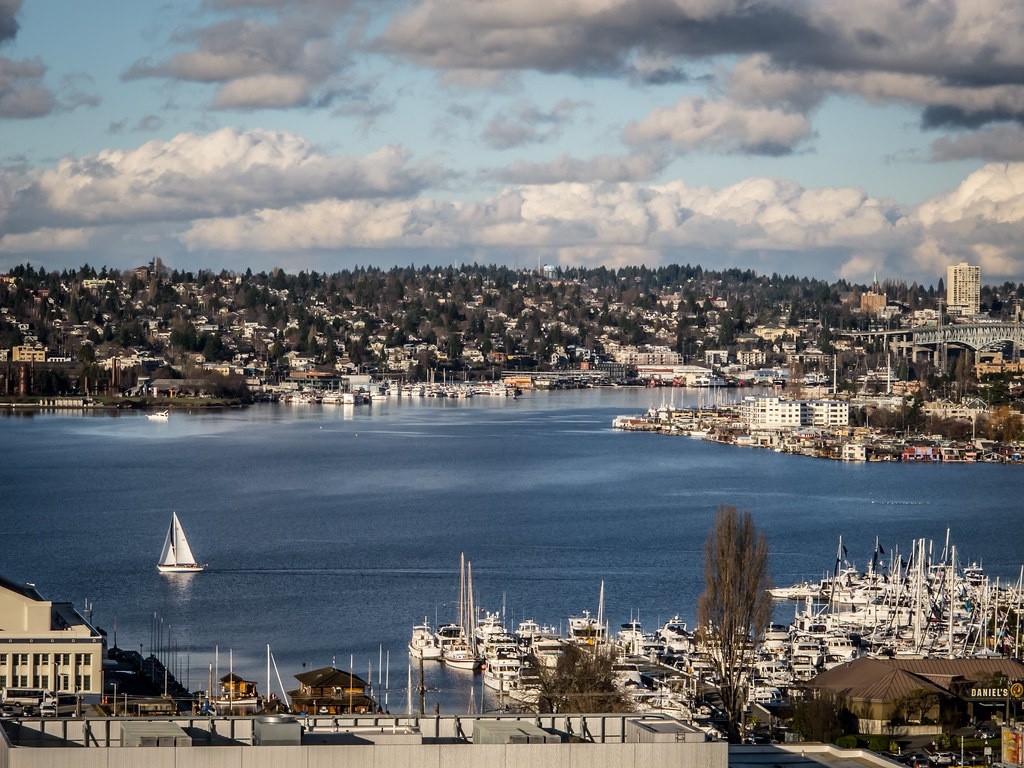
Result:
[767,583,827,602]
[694,622,796,707]
[156,511,204,574]
[472,610,545,703]
[530,579,711,720]
[386,380,507,399]
[785,527,1024,684]
[145,410,170,422]
[407,615,441,660]
[277,391,345,405]
[434,551,480,670]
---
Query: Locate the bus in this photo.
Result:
[2,686,59,708]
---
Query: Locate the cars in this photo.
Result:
[21,706,36,717]
[0,705,15,717]
[41,706,56,717]
